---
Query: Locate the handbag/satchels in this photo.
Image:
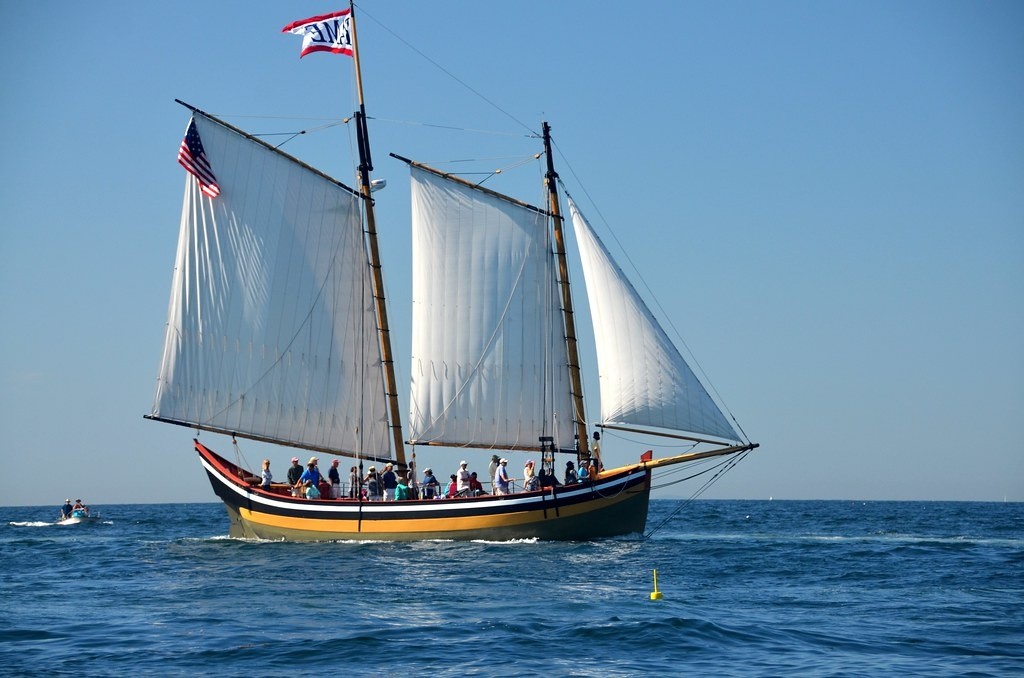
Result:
[425,484,435,497]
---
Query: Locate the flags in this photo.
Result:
[280,8,354,59]
[178,116,221,200]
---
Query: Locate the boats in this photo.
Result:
[56,508,101,526]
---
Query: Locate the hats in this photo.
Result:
[76,499,82,502]
[309,457,319,463]
[386,463,394,467]
[66,499,70,502]
[292,457,298,461]
[566,461,574,465]
[459,461,468,465]
[423,468,433,472]
[499,458,508,463]
[579,460,588,465]
[367,466,378,475]
[525,459,533,464]
[332,459,340,463]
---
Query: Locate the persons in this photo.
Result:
[565,461,578,485]
[524,460,535,481]
[421,467,440,500]
[457,461,473,498]
[61,499,83,518]
[328,459,341,498]
[348,466,357,499]
[590,431,601,475]
[576,460,590,484]
[261,459,272,492]
[287,457,304,496]
[489,454,517,496]
[291,463,319,498]
[365,461,419,502]
[443,472,482,499]
[308,457,324,481]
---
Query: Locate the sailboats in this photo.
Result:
[139,0,765,547]
